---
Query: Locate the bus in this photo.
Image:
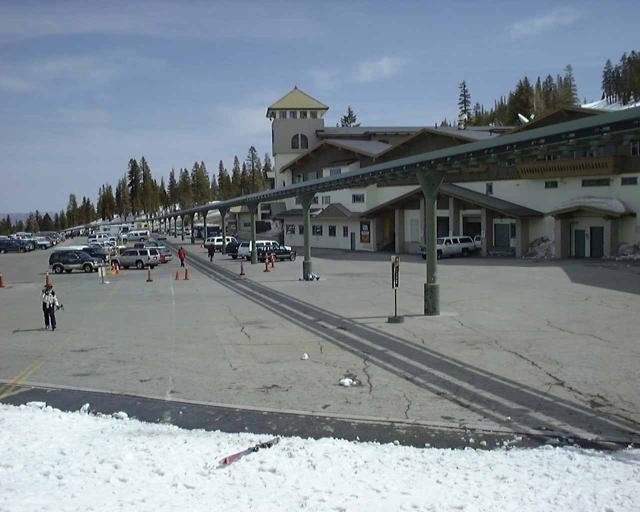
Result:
[193,224,221,238]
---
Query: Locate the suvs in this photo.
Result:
[110,249,160,269]
[49,249,105,273]
[226,239,296,261]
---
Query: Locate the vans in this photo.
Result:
[204,236,238,252]
[421,235,475,258]
[126,231,150,242]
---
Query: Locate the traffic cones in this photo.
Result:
[112,262,115,270]
[176,271,179,281]
[115,263,120,274]
[240,261,245,275]
[270,254,274,267]
[185,269,189,280]
[45,272,53,287]
[263,253,271,272]
[0,273,5,287]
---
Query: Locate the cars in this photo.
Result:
[143,246,173,263]
[88,224,128,259]
[59,229,86,238]
[474,234,482,248]
[0,232,62,253]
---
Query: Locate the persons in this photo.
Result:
[178,247,186,267]
[457,122,465,130]
[191,233,196,244]
[208,244,215,262]
[488,120,495,135]
[40,284,58,331]
